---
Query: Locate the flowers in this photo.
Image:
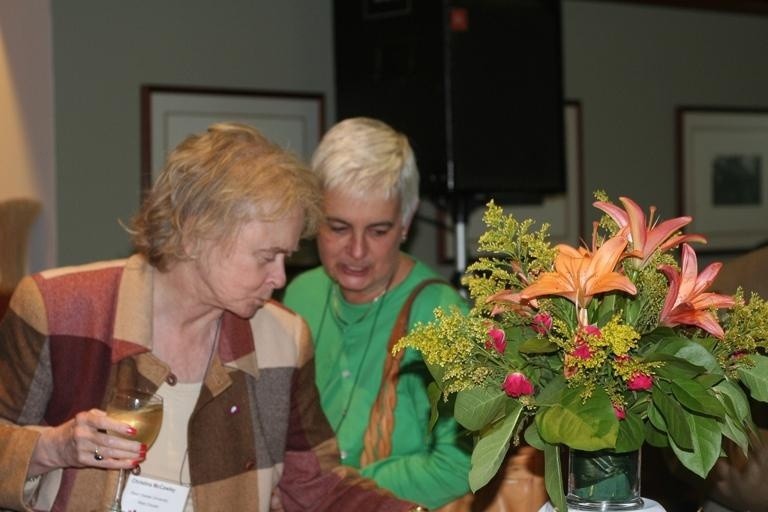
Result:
[391,189,768,480]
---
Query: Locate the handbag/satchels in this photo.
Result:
[427,444,549,510]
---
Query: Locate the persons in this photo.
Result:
[697,244,766,512]
[278,113,485,512]
[2,123,552,511]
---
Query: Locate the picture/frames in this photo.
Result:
[140,84,324,223]
[672,103,767,257]
[428,99,587,264]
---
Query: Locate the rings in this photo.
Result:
[93,449,105,460]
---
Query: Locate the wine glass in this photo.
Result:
[106,385,165,511]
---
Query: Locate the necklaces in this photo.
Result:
[159,298,204,385]
[329,280,382,332]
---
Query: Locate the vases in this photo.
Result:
[563,448,644,511]
